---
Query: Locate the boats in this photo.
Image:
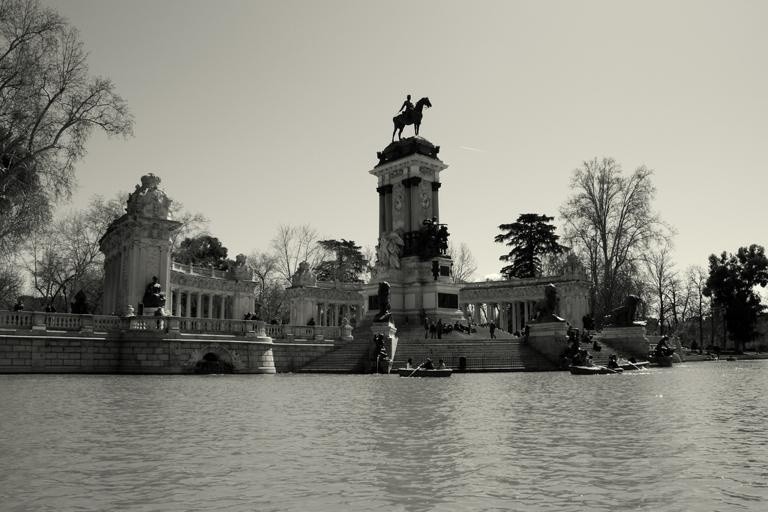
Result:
[615,362,651,369]
[566,364,623,375]
[397,368,453,377]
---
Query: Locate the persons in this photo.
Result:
[306,316,316,325]
[488,320,497,339]
[423,216,450,255]
[242,310,262,321]
[406,358,412,369]
[593,341,601,351]
[655,334,668,356]
[422,358,446,369]
[581,330,593,343]
[374,333,388,357]
[142,275,166,307]
[13,300,24,311]
[46,303,56,312]
[424,317,477,339]
[399,95,414,117]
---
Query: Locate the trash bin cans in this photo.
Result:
[459,356,466,368]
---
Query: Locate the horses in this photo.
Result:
[391,97,432,143]
